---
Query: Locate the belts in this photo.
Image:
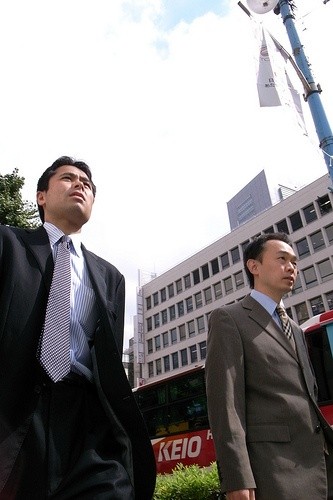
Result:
[62,375,86,384]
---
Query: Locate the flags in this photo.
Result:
[255,25,308,136]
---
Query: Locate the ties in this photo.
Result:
[276,305,330,457]
[39,236,72,384]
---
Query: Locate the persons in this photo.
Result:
[0,156,155,500]
[205,234,332,500]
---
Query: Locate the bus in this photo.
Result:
[129,308,333,477]
